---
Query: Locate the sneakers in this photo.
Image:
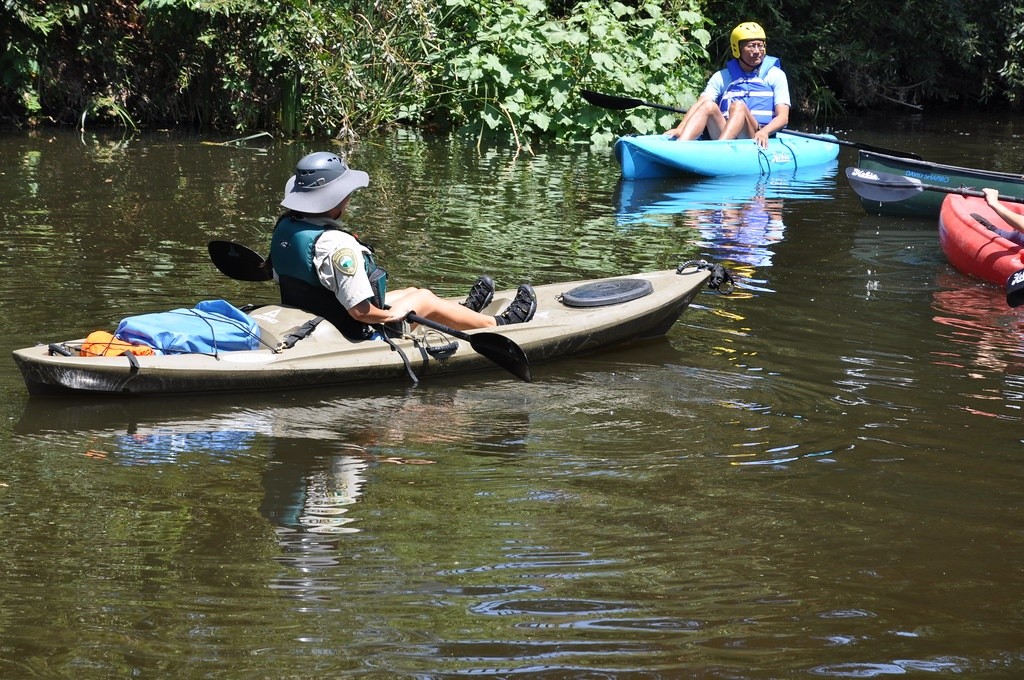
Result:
[458,275,495,314]
[501,285,537,325]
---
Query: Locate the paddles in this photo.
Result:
[845,165,1023,206]
[580,88,921,159]
[207,240,532,384]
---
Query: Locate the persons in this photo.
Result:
[271,152,537,341]
[663,22,791,150]
[970,187,1024,249]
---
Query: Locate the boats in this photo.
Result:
[610,130,844,179]
[7,256,718,399]
[851,148,1024,220]
[938,191,1024,286]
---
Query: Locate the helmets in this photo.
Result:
[280,152,369,215]
[731,21,766,60]
